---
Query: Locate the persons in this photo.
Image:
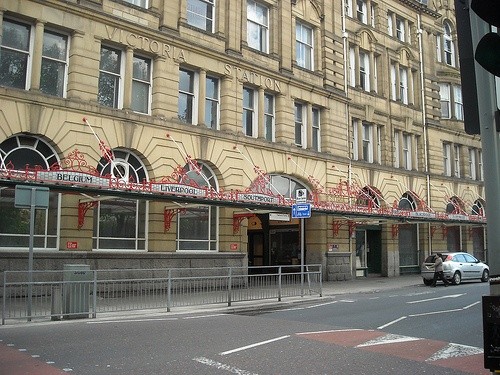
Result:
[429,252,450,288]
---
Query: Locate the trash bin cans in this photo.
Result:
[62,263,90,319]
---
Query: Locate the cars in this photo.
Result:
[420,252,489,285]
